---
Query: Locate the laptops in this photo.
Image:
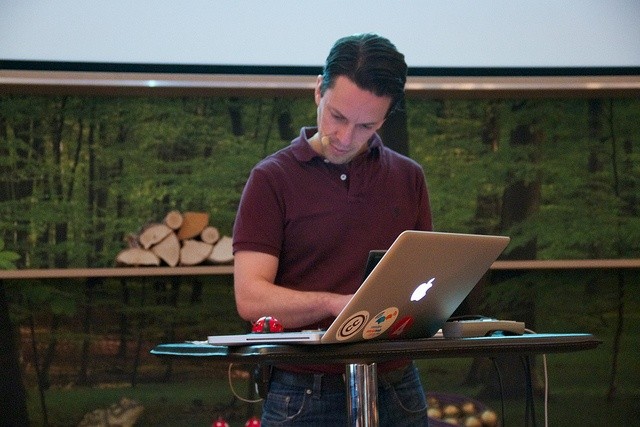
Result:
[207,230,510,345]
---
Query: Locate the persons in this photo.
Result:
[232,33,432,426]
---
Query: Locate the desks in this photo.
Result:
[149,329,603,426]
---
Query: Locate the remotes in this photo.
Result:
[443,319,526,338]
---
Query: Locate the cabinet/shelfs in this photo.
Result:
[0,68,640,277]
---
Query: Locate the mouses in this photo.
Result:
[251,316,284,333]
